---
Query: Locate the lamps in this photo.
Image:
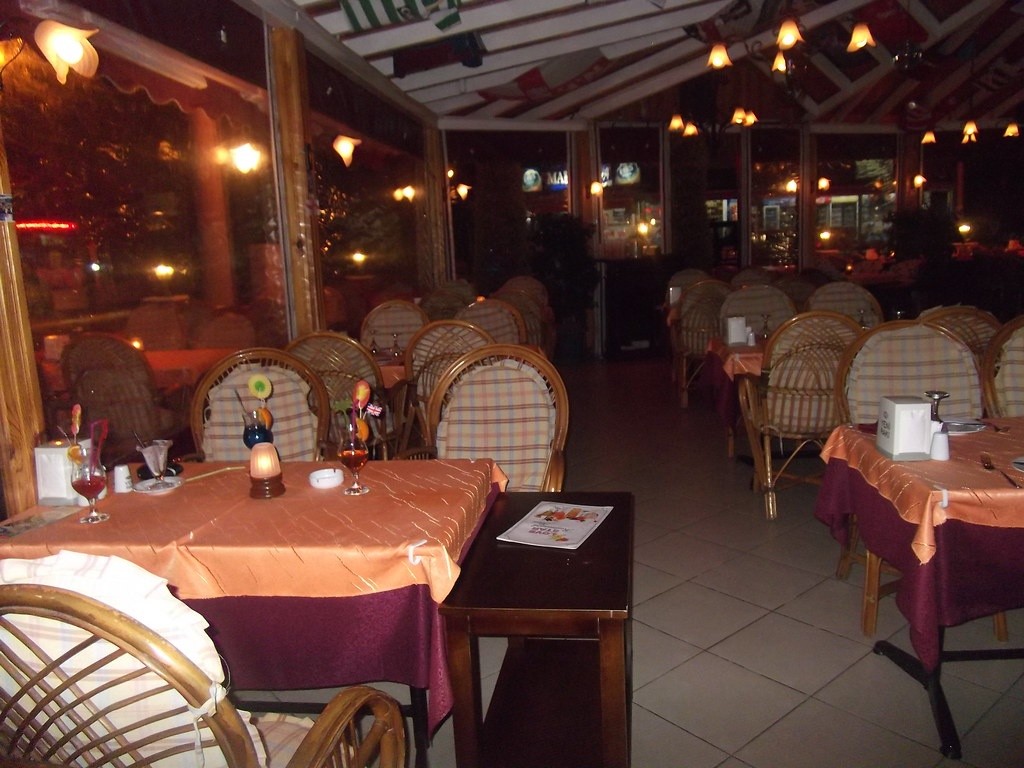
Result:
[668,0,1024,154]
[0,15,99,91]
[304,133,362,173]
[442,182,472,202]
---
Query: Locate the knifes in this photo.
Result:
[1012,461,1024,465]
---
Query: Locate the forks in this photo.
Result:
[975,418,1008,432]
[980,453,1023,489]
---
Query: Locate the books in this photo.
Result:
[495,500,614,550]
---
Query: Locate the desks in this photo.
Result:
[1,459,510,766]
[815,417,1024,758]
[438,490,636,768]
[702,338,821,490]
[370,350,405,388]
[36,349,239,410]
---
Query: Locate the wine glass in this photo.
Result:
[71,447,111,524]
[924,391,950,433]
[389,330,403,366]
[856,308,872,331]
[336,424,369,496]
[756,314,774,345]
[367,329,380,361]
[135,440,175,490]
[241,410,274,477]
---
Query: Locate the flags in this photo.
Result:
[366,404,382,416]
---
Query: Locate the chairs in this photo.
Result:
[0,551,407,766]
[665,269,1024,639]
[61,302,255,470]
[192,276,569,492]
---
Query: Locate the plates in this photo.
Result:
[133,477,183,496]
[944,421,985,436]
[1012,455,1024,472]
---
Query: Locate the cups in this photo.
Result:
[930,432,949,461]
[891,307,907,320]
[748,332,755,346]
[113,464,133,492]
